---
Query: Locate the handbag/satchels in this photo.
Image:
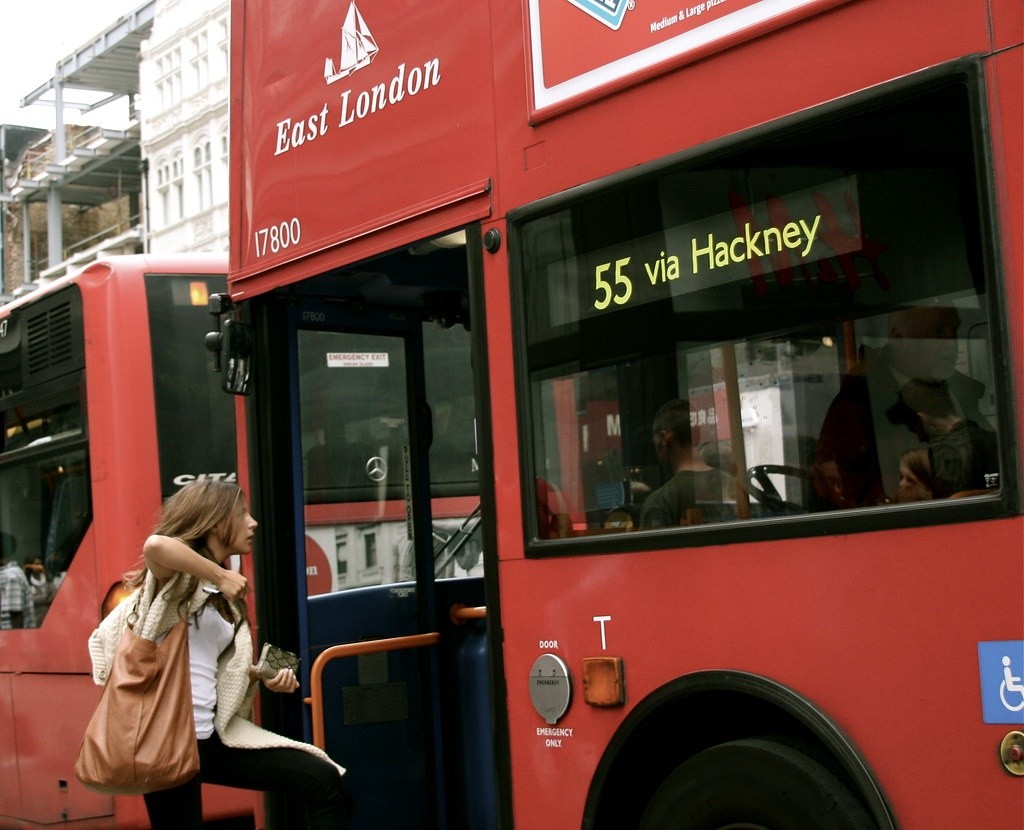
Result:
[76,537,200,795]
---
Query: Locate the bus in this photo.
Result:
[0,250,581,830]
[204,0,1024,830]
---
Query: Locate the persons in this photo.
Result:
[634,398,757,536]
[89,479,352,830]
[0,533,58,630]
[806,300,1001,529]
